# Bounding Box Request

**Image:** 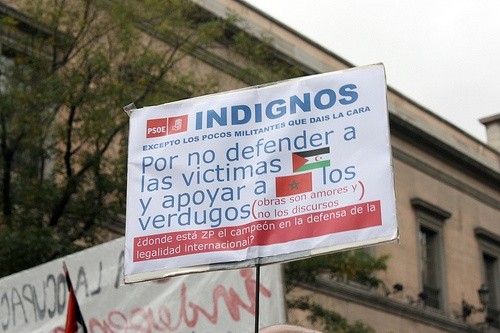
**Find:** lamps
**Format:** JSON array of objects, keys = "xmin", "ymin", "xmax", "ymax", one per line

[{"xmin": 462, "ymin": 283, "xmax": 490, "ymax": 320}]
